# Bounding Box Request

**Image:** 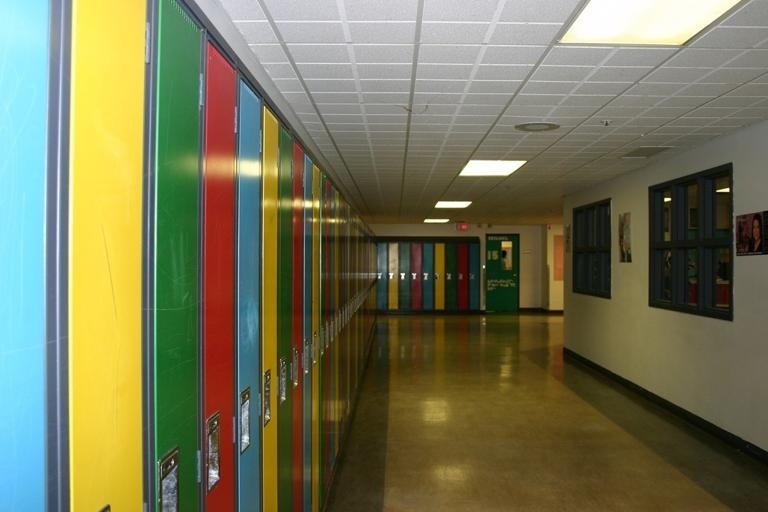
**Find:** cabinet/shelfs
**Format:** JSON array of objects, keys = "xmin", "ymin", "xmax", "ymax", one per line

[
  {"xmin": 377, "ymin": 242, "xmax": 482, "ymax": 313},
  {"xmin": 3, "ymin": 2, "xmax": 377, "ymax": 511}
]
